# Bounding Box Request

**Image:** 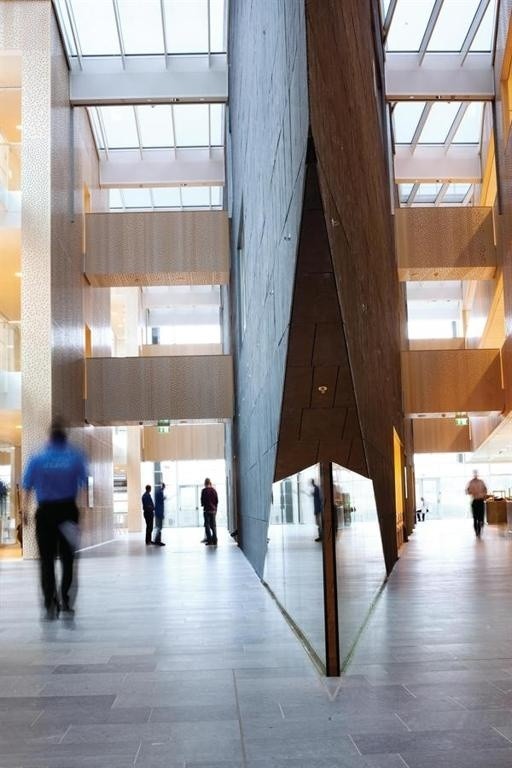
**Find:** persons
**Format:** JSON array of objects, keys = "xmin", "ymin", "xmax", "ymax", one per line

[
  {"xmin": 21, "ymin": 419, "xmax": 88, "ymax": 620},
  {"xmin": 311, "ymin": 480, "xmax": 325, "ymax": 542},
  {"xmin": 201, "ymin": 478, "xmax": 218, "ymax": 544},
  {"xmin": 142, "ymin": 485, "xmax": 155, "ymax": 545},
  {"xmin": 421, "ymin": 497, "xmax": 426, "ymax": 521},
  {"xmin": 465, "ymin": 470, "xmax": 487, "ymax": 537},
  {"xmin": 154, "ymin": 482, "xmax": 165, "ymax": 547}
]
